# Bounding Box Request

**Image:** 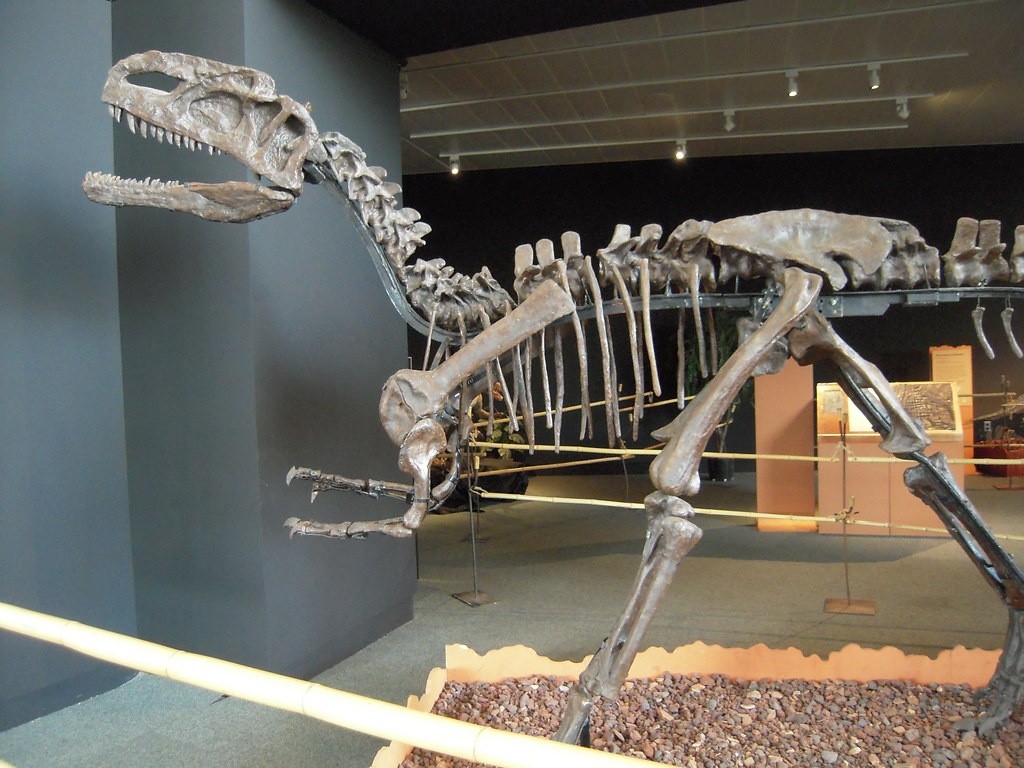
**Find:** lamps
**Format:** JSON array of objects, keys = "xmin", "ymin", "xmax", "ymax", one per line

[
  {"xmin": 675, "ymin": 139, "xmax": 688, "ymax": 159},
  {"xmin": 896, "ymin": 98, "xmax": 910, "ymax": 119},
  {"xmin": 449, "ymin": 154, "xmax": 461, "ymax": 175},
  {"xmin": 866, "ymin": 64, "xmax": 881, "ymax": 91},
  {"xmin": 723, "ymin": 111, "xmax": 736, "ymax": 131},
  {"xmin": 785, "ymin": 71, "xmax": 799, "ymax": 97},
  {"xmin": 399, "ymin": 70, "xmax": 409, "ymax": 99}
]
